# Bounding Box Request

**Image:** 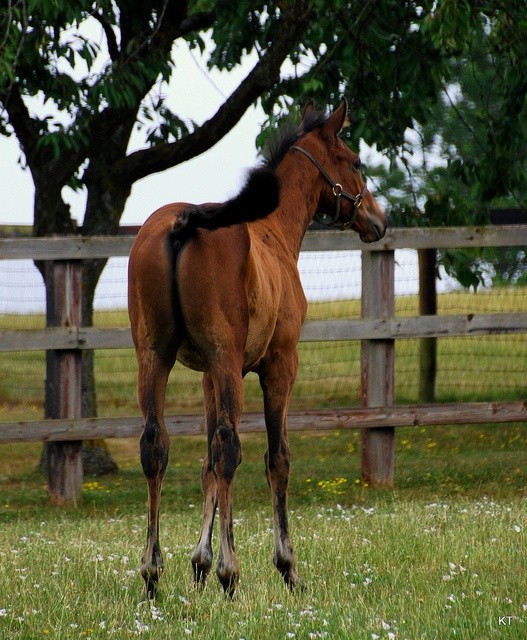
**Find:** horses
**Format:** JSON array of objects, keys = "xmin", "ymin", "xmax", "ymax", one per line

[{"xmin": 126, "ymin": 94, "xmax": 390, "ymax": 597}]
